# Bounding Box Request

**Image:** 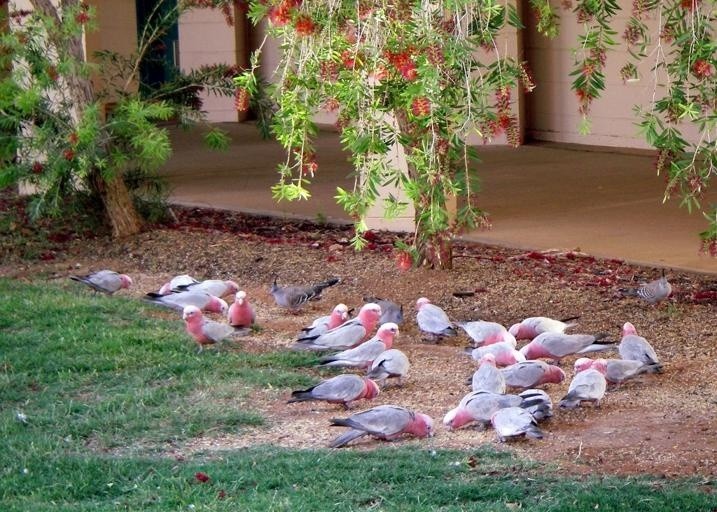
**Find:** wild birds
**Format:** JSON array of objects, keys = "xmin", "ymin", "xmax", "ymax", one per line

[
  {"xmin": 143, "ymin": 272, "xmax": 257, "ymax": 348},
  {"xmin": 615, "ymin": 275, "xmax": 674, "ymax": 309},
  {"xmin": 497, "ymin": 358, "xmax": 567, "ymax": 391},
  {"xmin": 448, "ymin": 314, "xmax": 517, "ymax": 350},
  {"xmin": 571, "ymin": 357, "xmax": 654, "ymax": 396},
  {"xmin": 267, "ymin": 277, "xmax": 338, "ymax": 315},
  {"xmin": 69, "ymin": 270, "xmax": 134, "ymax": 297},
  {"xmin": 366, "ymin": 348, "xmax": 412, "ymax": 394},
  {"xmin": 296, "ymin": 311, "xmax": 353, "ymax": 343},
  {"xmin": 327, "ymin": 403, "xmax": 432, "ymax": 449},
  {"xmin": 288, "ymin": 302, "xmax": 382, "ymax": 357},
  {"xmin": 416, "ymin": 298, "xmax": 460, "ymax": 344},
  {"xmin": 285, "ymin": 372, "xmax": 380, "ymax": 411},
  {"xmin": 553, "ymin": 356, "xmax": 607, "ymax": 413},
  {"xmin": 441, "ymin": 387, "xmax": 553, "ymax": 429},
  {"xmin": 301, "ymin": 301, "xmax": 355, "ymax": 336},
  {"xmin": 377, "ymin": 301, "xmax": 405, "ymax": 330},
  {"xmin": 616, "ymin": 323, "xmax": 663, "ymax": 375},
  {"xmin": 508, "ymin": 314, "xmax": 574, "ymax": 341},
  {"xmin": 473, "ymin": 358, "xmax": 507, "ymax": 399},
  {"xmin": 465, "ymin": 339, "xmax": 527, "ymax": 367},
  {"xmin": 293, "ymin": 322, "xmax": 401, "ymax": 371},
  {"xmin": 518, "ymin": 330, "xmax": 620, "ymax": 367},
  {"xmin": 489, "ymin": 405, "xmax": 543, "ymax": 442}
]
